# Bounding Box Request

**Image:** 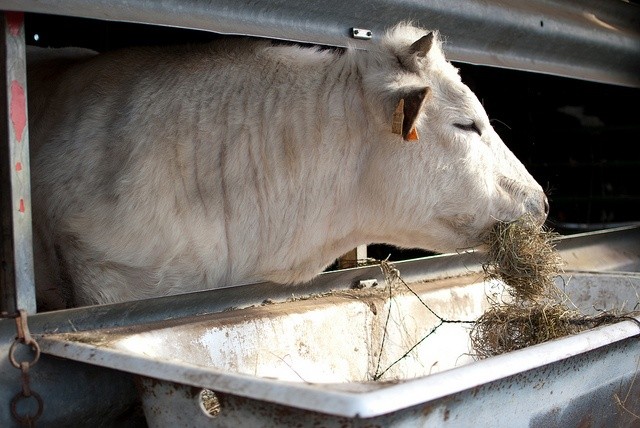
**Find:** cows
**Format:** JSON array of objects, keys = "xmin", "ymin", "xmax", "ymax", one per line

[{"xmin": 24, "ymin": 17, "xmax": 550, "ymax": 312}]
